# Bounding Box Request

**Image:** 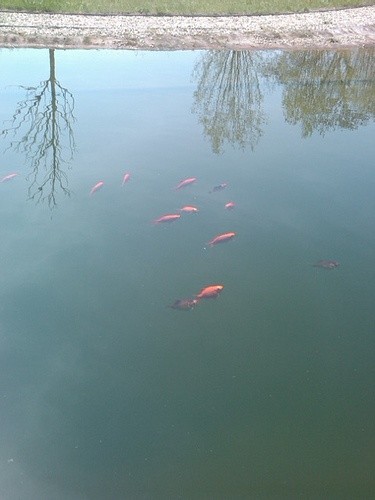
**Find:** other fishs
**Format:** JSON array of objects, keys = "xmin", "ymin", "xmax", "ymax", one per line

[
  {"xmin": 172, "ymin": 177, "xmax": 197, "ymax": 192},
  {"xmin": 166, "ymin": 299, "xmax": 199, "ymax": 312},
  {"xmin": 0, "ymin": 173, "xmax": 17, "ymax": 184},
  {"xmin": 193, "ymin": 285, "xmax": 224, "ymax": 301},
  {"xmin": 224, "ymin": 202, "xmax": 240, "ymax": 209},
  {"xmin": 208, "ymin": 182, "xmax": 227, "ymax": 195},
  {"xmin": 312, "ymin": 259, "xmax": 340, "ymax": 272},
  {"xmin": 176, "ymin": 205, "xmax": 200, "ymax": 216},
  {"xmin": 120, "ymin": 172, "xmax": 131, "ymax": 186},
  {"xmin": 88, "ymin": 181, "xmax": 104, "ymax": 196},
  {"xmin": 150, "ymin": 214, "xmax": 182, "ymax": 226},
  {"xmin": 207, "ymin": 231, "xmax": 236, "ymax": 248}
]
